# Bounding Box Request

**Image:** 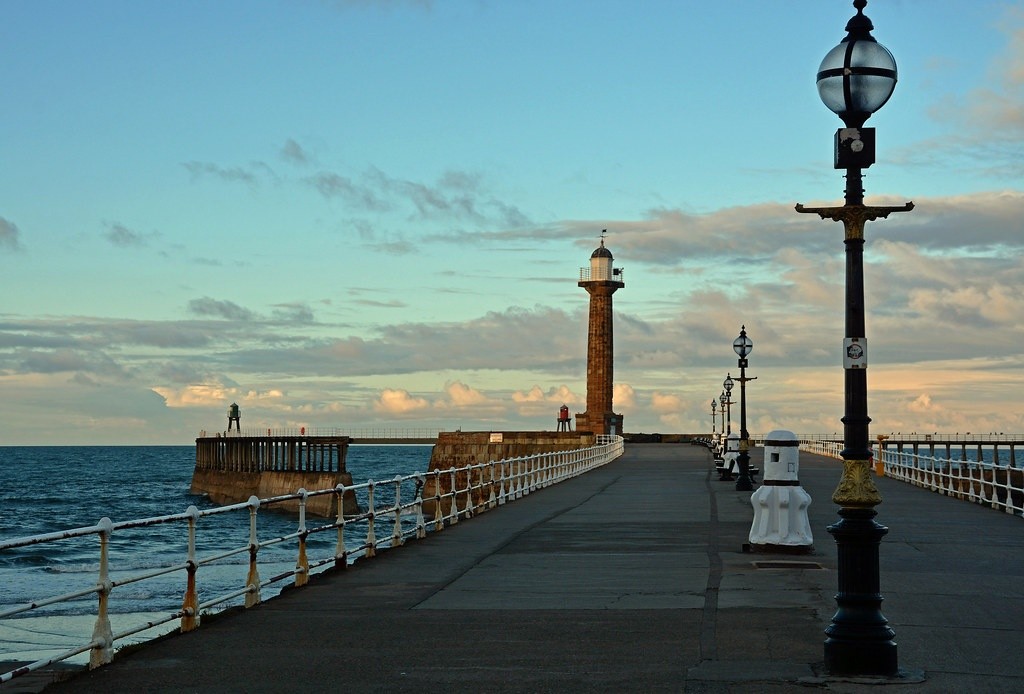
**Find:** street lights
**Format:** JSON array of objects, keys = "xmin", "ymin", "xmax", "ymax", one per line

[
  {"xmin": 720, "ymin": 390, "xmax": 726, "ymax": 433},
  {"xmin": 710, "ymin": 398, "xmax": 718, "ymax": 433},
  {"xmin": 728, "ymin": 325, "xmax": 756, "ymax": 492},
  {"xmin": 813, "ymin": 1, "xmax": 904, "ymax": 675},
  {"xmin": 723, "ymin": 372, "xmax": 734, "ymax": 449}
]
